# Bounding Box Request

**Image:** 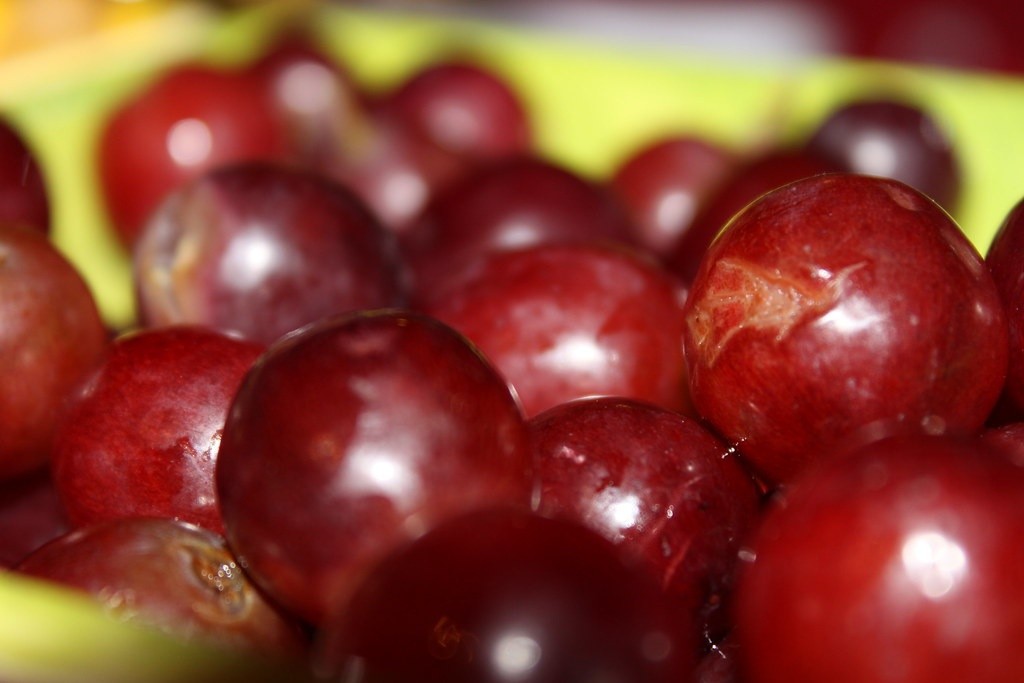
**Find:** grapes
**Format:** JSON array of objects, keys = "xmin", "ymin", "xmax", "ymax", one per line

[{"xmin": 1, "ymin": 21, "xmax": 1023, "ymax": 682}]
